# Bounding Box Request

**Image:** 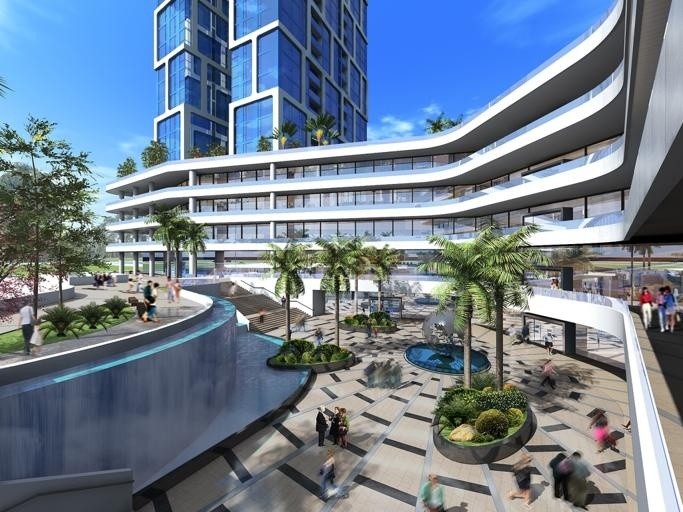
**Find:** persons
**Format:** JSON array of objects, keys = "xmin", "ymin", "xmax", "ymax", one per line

[
  {"xmin": 315, "ymin": 327, "xmax": 324, "ymax": 346},
  {"xmin": 551, "ymin": 278, "xmax": 560, "ymax": 290},
  {"xmin": 419, "ymin": 473, "xmax": 447, "ymax": 512},
  {"xmin": 509, "ymin": 323, "xmax": 560, "ymax": 389},
  {"xmin": 17, "ymin": 269, "xmax": 183, "ymax": 358},
  {"xmin": 363, "ymin": 359, "xmax": 403, "ymax": 389},
  {"xmin": 319, "ymin": 448, "xmax": 344, "ymax": 502},
  {"xmin": 314, "ymin": 404, "xmax": 351, "ymax": 450},
  {"xmin": 505, "ymin": 409, "xmax": 632, "ymax": 511},
  {"xmin": 638, "ymin": 285, "xmax": 680, "ymax": 334}
]
